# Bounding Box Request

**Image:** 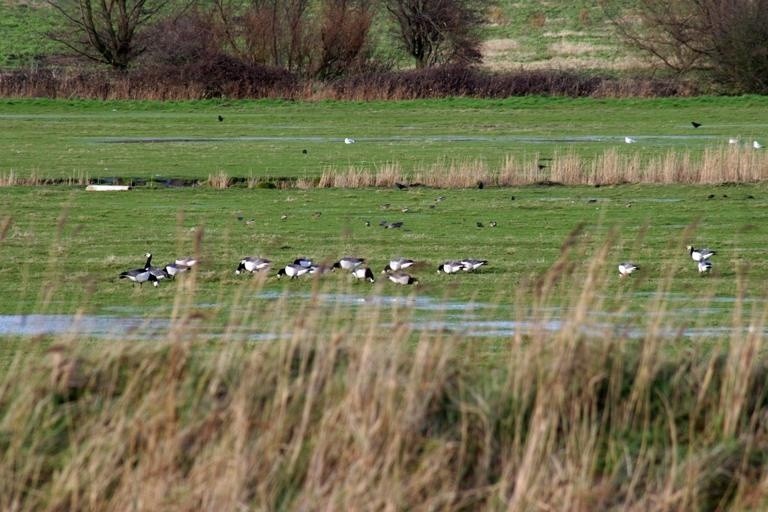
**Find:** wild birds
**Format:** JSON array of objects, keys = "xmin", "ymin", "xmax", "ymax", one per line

[
  {"xmin": 707, "ymin": 193, "xmax": 754, "ymax": 199},
  {"xmin": 625, "ymin": 136, "xmax": 636, "ymax": 143},
  {"xmin": 303, "ymin": 149, "xmax": 307, "ymax": 154},
  {"xmin": 345, "ymin": 138, "xmax": 356, "ymax": 145},
  {"xmin": 218, "ymin": 115, "xmax": 224, "ymax": 122},
  {"xmin": 237, "ymin": 181, "xmax": 516, "ymax": 229},
  {"xmin": 236, "ymin": 256, "xmax": 489, "ymax": 288},
  {"xmin": 588, "ymin": 199, "xmax": 597, "ymax": 203},
  {"xmin": 626, "ymin": 200, "xmax": 632, "ymax": 208},
  {"xmin": 617, "ymin": 262, "xmax": 641, "ymax": 278},
  {"xmin": 118, "ymin": 253, "xmax": 198, "ymax": 289},
  {"xmin": 691, "ymin": 121, "xmax": 702, "ymax": 128},
  {"xmin": 697, "ymin": 256, "xmax": 712, "ymax": 276},
  {"xmin": 685, "ymin": 244, "xmax": 716, "ymax": 262},
  {"xmin": 752, "ymin": 140, "xmax": 764, "ymax": 149},
  {"xmin": 729, "ymin": 133, "xmax": 741, "ymax": 144}
]
